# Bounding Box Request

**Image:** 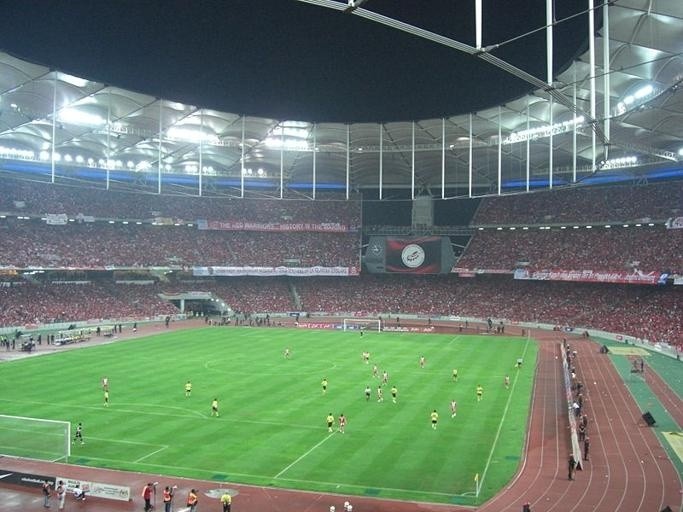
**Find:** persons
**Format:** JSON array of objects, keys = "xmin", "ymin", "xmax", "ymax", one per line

[
  {"xmin": 163, "ymin": 486, "xmax": 173, "ymax": 512},
  {"xmin": 187, "ymin": 487, "xmax": 198, "ymax": 512},
  {"xmin": 1, "ymin": 174, "xmax": 683, "ymax": 482},
  {"xmin": 143, "ymin": 482, "xmax": 152, "ymax": 512},
  {"xmin": 73, "ymin": 484, "xmax": 86, "ymax": 501},
  {"xmin": 42, "ymin": 479, "xmax": 50, "ymax": 508},
  {"xmin": 523, "ymin": 501, "xmax": 530, "ymax": 512},
  {"xmin": 56, "ymin": 481, "xmax": 66, "ymax": 510},
  {"xmin": 220, "ymin": 491, "xmax": 232, "ymax": 512}
]
